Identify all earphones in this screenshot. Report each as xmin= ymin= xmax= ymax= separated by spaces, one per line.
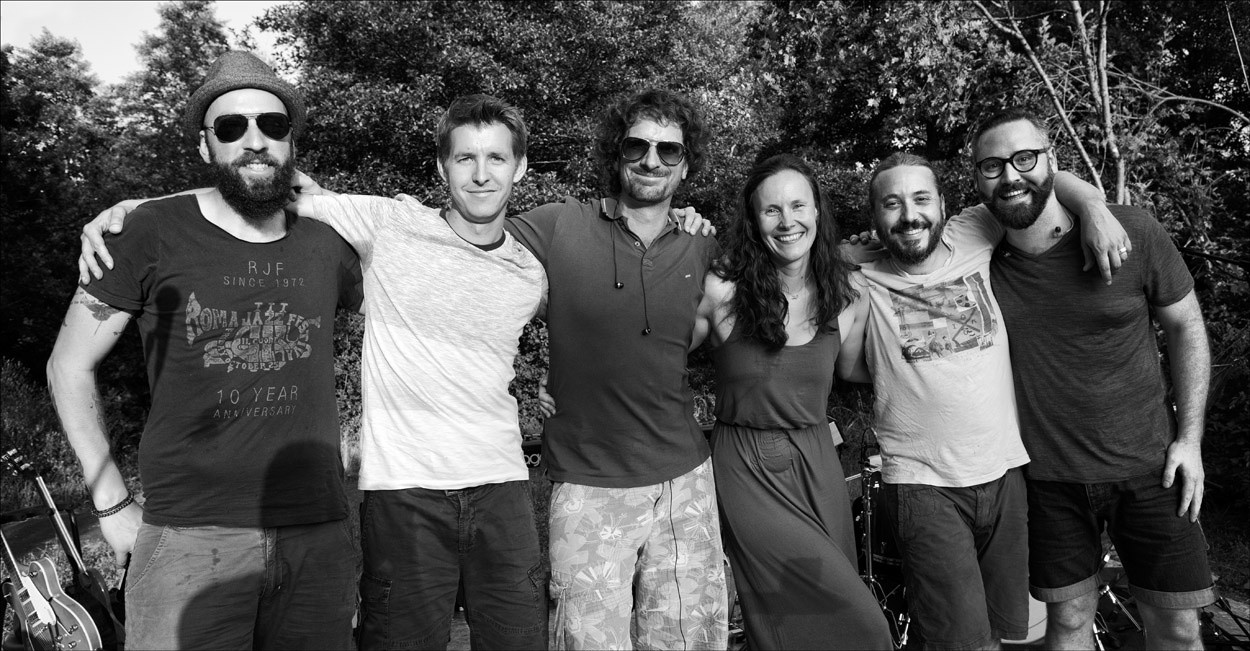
xmin=642 ymin=328 xmax=651 ymax=335
xmin=615 ymin=282 xmax=624 ymax=289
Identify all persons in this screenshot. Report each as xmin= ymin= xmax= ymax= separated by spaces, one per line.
xmin=841 ymin=105 xmax=1221 ymax=651
xmin=289 ymin=91 xmax=892 ymax=651
xmin=78 ymin=92 xmax=718 ymax=651
xmin=537 ymin=152 xmax=895 ymax=651
xmin=46 ymin=50 xmax=365 ymax=650
xmin=671 ymin=152 xmax=1133 ymax=651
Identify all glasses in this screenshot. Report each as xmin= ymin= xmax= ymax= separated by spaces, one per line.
xmin=976 ymin=148 xmax=1046 ymax=180
xmin=202 ymin=112 xmax=292 ymax=143
xmin=618 ymin=136 xmax=688 ymax=166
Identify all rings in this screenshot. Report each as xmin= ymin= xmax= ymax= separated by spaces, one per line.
xmin=1118 ymin=248 xmax=1127 ymax=254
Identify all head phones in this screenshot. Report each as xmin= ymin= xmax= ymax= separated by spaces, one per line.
xmin=999 ymin=201 xmax=1075 ymax=257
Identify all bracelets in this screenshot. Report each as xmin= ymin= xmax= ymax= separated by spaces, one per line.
xmin=90 ymin=489 xmax=134 ymax=518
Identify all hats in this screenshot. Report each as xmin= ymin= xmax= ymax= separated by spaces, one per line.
xmin=185 ymin=50 xmax=307 ymax=145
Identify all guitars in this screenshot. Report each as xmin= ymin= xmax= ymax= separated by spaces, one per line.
xmin=1 ymin=445 xmax=125 ymax=651
xmin=0 ymin=529 xmax=103 ymax=651
